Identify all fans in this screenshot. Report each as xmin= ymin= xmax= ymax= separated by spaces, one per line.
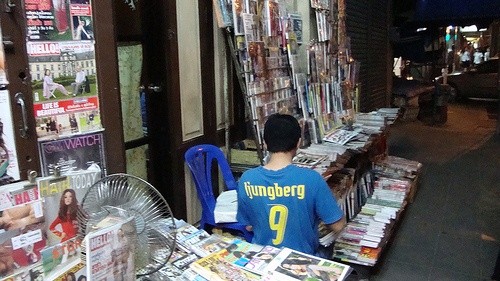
xmin=75 ymin=172 xmax=177 ymax=279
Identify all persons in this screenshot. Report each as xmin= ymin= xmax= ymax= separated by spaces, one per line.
xmin=50 ymin=189 xmax=90 ymax=243
xmin=43 ymin=65 xmax=86 ymax=98
xmin=448 ymin=45 xmax=490 ymax=72
xmin=279 ymin=257 xmax=343 ymax=281
xmin=236 ymin=113 xmax=346 ymax=257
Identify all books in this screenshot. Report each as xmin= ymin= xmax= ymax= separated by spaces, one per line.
xmin=0 ymin=178 xmax=354 ymax=281
xmin=222 ymin=0 xmax=423 ymax=267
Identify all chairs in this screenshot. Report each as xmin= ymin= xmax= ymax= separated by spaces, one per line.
xmin=186 ymin=144 xmax=254 ymax=244
